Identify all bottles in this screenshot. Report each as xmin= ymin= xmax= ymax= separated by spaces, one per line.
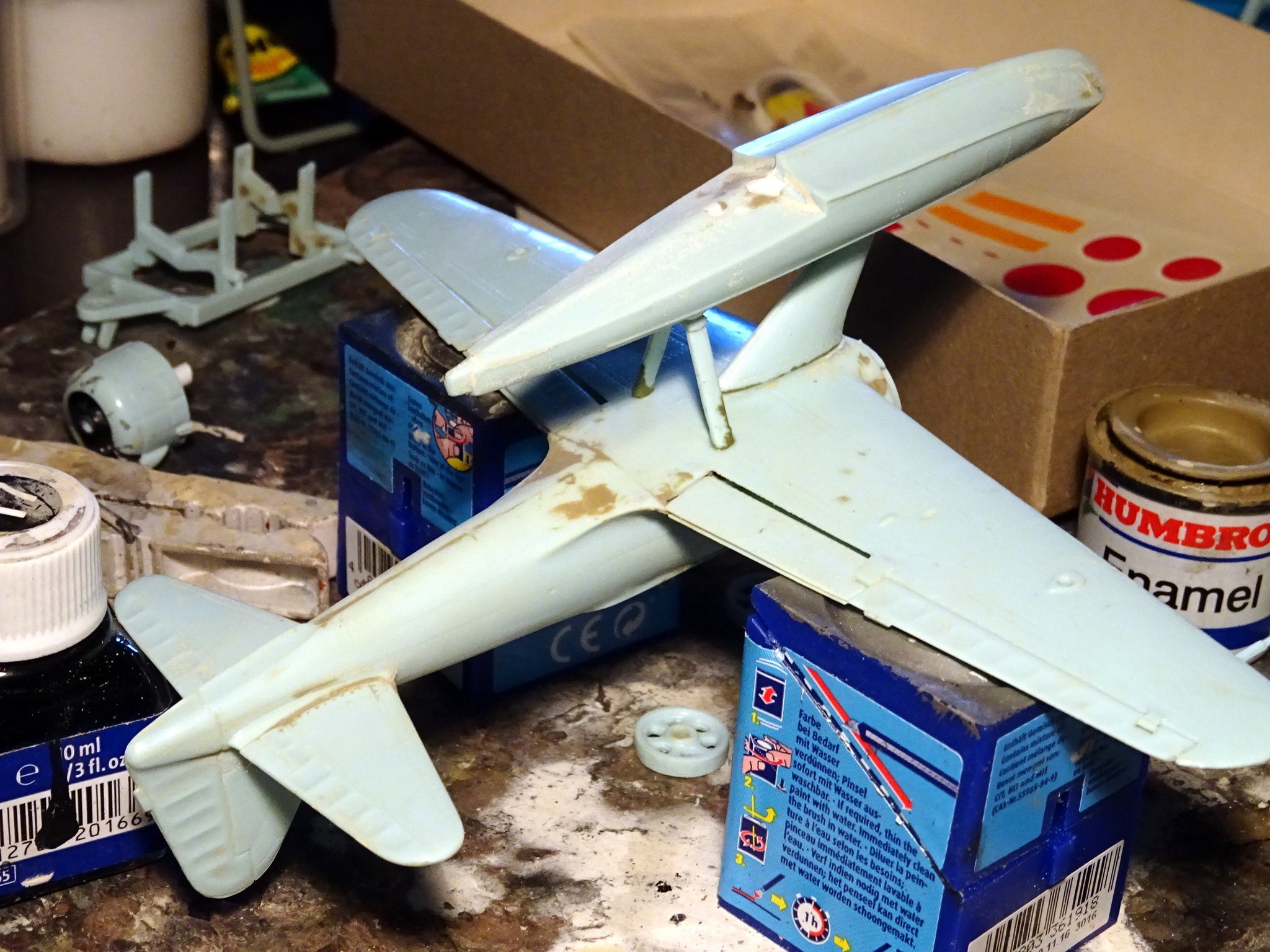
xmin=0 ymin=462 xmax=180 ymax=907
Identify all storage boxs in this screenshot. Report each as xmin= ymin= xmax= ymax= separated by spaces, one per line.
xmin=335 ymin=0 xmax=1270 ymax=515
xmin=335 ymin=300 xmax=716 ymax=696
xmin=717 ymin=572 xmax=1147 ymax=951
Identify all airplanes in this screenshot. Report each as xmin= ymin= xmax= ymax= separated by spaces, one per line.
xmin=111 ymin=47 xmax=1268 ymax=903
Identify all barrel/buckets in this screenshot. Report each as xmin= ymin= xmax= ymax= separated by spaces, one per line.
xmin=1075 ymin=381 xmax=1270 ymax=654
